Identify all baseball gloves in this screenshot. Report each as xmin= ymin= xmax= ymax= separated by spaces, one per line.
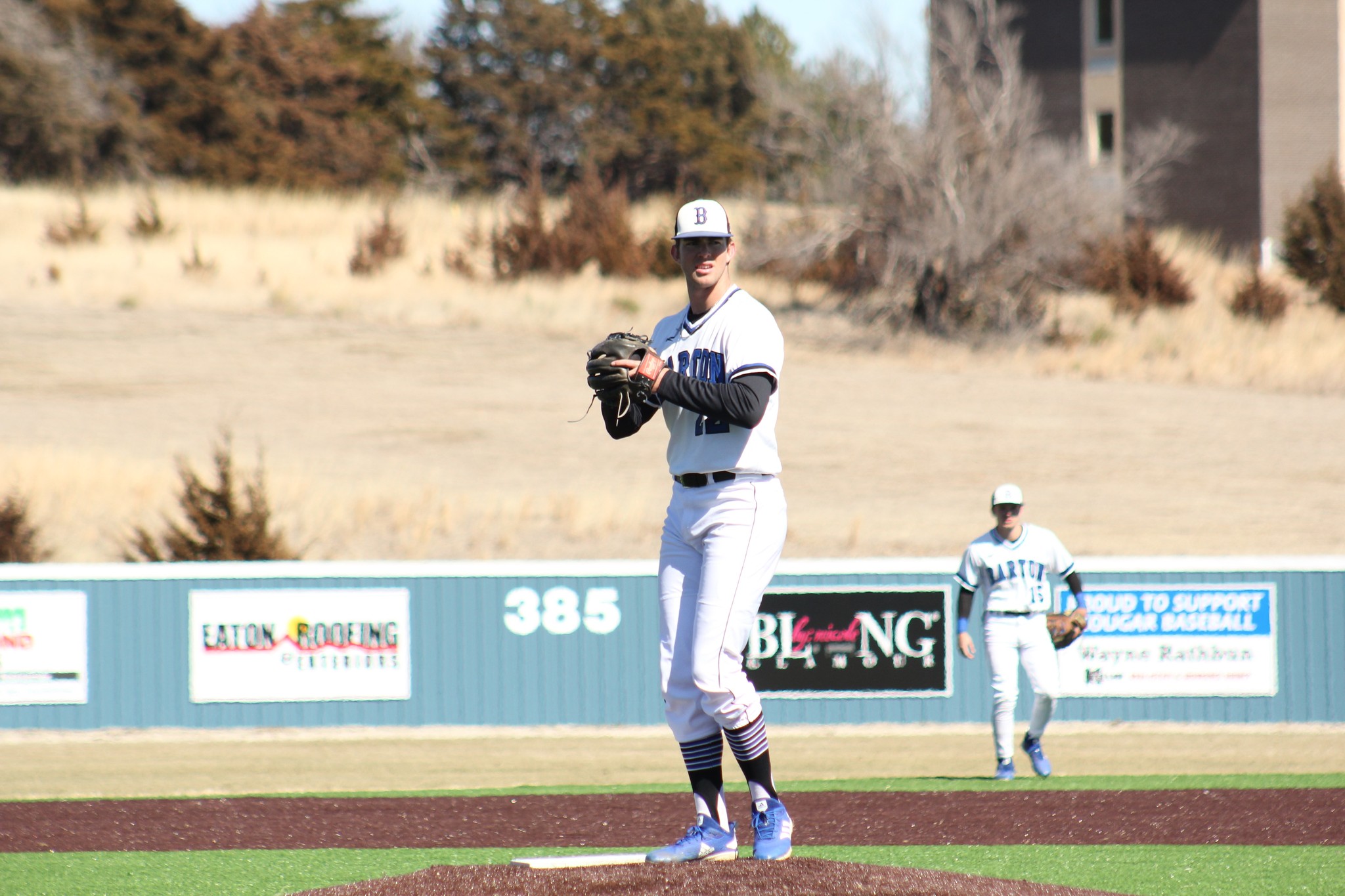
xmin=567 ymin=326 xmax=667 ymax=425
xmin=1046 ymin=610 xmax=1089 ymax=650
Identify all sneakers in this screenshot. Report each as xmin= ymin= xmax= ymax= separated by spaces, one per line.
xmin=752 ymin=798 xmax=792 ymax=861
xmin=1023 ymin=732 xmax=1052 ymax=777
xmin=996 ymin=759 xmax=1014 ymax=782
xmin=646 ymin=813 xmax=739 ymax=862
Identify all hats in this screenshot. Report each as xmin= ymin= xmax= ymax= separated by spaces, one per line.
xmin=993 ymin=483 xmax=1024 ymax=506
xmin=671 ymin=199 xmax=734 ymax=238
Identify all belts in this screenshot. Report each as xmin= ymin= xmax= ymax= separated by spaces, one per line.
xmin=991 ymin=611 xmax=1031 ymax=616
xmin=674 ymin=471 xmax=735 ymax=488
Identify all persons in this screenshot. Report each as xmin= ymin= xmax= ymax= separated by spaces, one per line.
xmin=569 ymin=200 xmax=796 ymax=866
xmin=955 ymin=483 xmax=1088 ymax=782
